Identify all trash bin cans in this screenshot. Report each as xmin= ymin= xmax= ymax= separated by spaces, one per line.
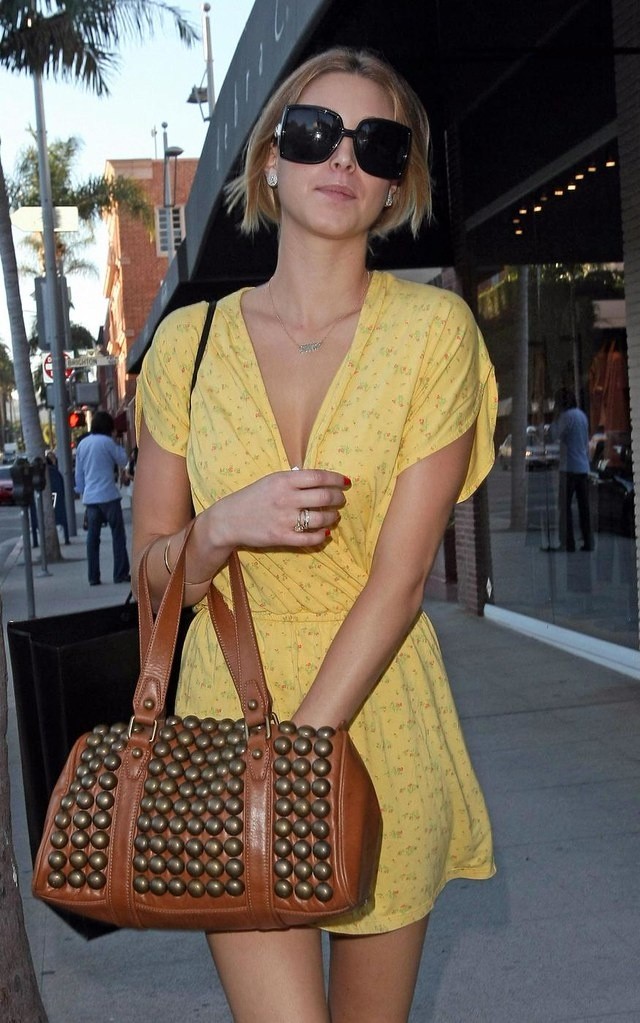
xmin=525 ymin=459 xmax=559 ymax=531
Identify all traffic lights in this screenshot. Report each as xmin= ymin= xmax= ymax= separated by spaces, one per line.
xmin=69 ymin=412 xmax=87 ymax=428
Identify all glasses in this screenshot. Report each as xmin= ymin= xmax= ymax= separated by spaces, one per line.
xmin=276 ymin=104 xmax=413 ymax=185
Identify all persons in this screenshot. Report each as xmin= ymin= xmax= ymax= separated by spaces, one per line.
xmin=131 ymin=50 xmax=497 ymax=1023
xmin=543 ymin=388 xmax=594 ymax=552
xmin=74 ymin=412 xmax=132 ymax=586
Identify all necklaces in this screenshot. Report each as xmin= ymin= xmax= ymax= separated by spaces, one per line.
xmin=268 ymin=269 xmax=369 ymax=355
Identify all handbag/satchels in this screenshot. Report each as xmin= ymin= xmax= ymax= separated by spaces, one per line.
xmin=8 ymin=601 xmax=202 ymax=943
xmin=27 ymin=518 xmax=389 ymax=939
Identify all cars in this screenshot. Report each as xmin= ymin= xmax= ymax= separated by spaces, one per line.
xmin=497 ymin=424 xmax=559 ymax=473
xmin=580 ymin=430 xmax=637 ymax=542
xmin=0 ymin=442 xmax=26 ymax=507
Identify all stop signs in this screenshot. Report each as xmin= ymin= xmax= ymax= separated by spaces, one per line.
xmin=43 ymin=352 xmax=74 ymax=381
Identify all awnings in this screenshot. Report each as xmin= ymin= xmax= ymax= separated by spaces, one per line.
xmin=45 ymin=382 xmax=98 ymax=449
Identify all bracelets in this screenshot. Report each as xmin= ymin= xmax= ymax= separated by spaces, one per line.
xmin=164 ymin=534 xmax=194 ymax=585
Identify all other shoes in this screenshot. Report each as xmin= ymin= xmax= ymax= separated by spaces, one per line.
xmin=113 ymin=574 xmax=131 ymax=584
xmin=89 ymin=580 xmax=101 ymax=586
xmin=552 ymin=542 xmax=576 ymax=552
xmin=579 ymin=543 xmax=595 ymax=551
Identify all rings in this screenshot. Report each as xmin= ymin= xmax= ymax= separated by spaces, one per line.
xmin=295 ymin=509 xmax=309 ymax=532
